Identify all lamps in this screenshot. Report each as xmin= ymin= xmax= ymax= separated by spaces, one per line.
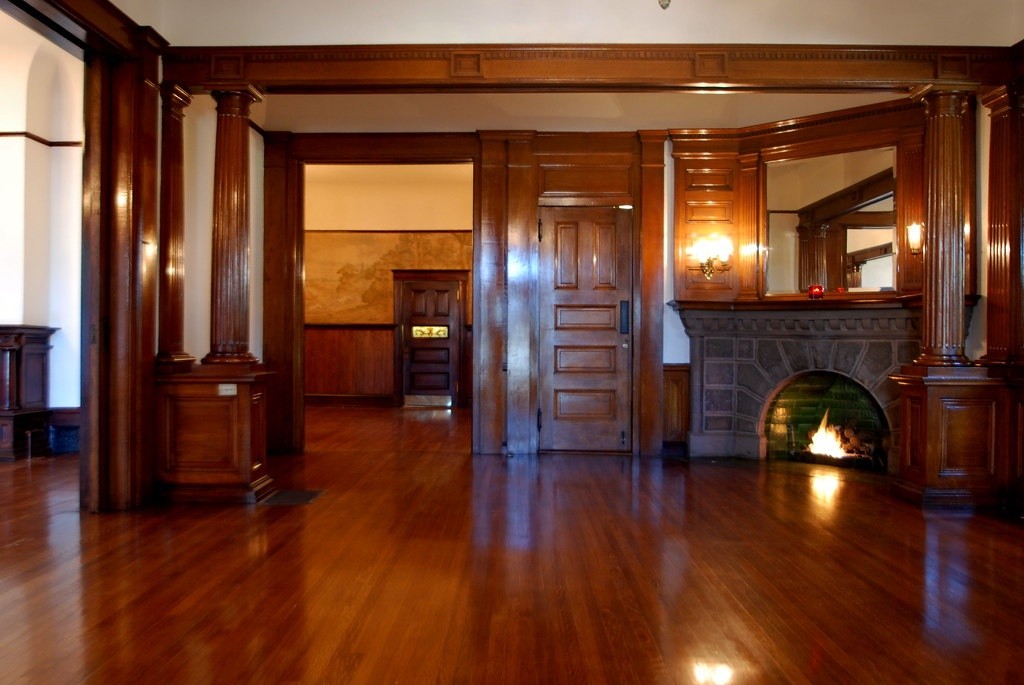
xmin=907 ymin=222 xmax=925 ymax=266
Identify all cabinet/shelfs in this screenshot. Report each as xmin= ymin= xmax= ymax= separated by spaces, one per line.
xmin=0 ymin=324 xmax=60 ymax=461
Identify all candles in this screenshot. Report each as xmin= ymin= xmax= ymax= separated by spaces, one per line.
xmin=808 ymin=285 xmax=824 ymax=300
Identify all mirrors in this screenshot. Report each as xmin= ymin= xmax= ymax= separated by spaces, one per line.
xmin=763 ymin=144 xmax=899 ymax=296
